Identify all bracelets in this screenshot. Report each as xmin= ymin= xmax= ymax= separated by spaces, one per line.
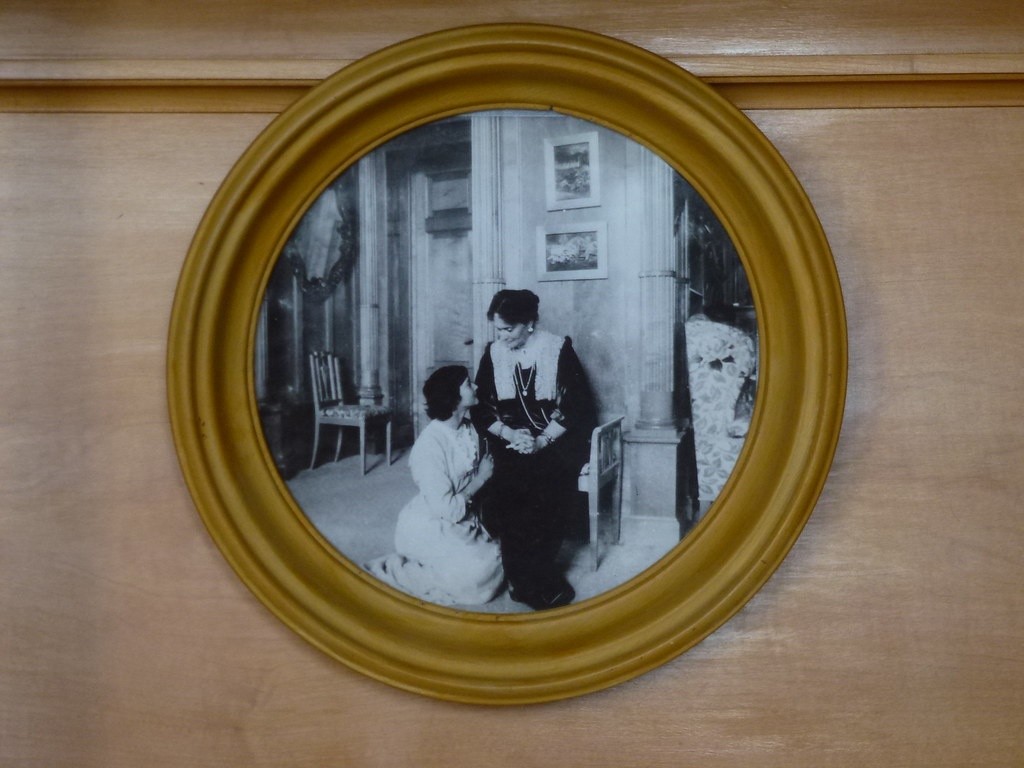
xmin=542 ymin=431 xmax=556 ymax=444
xmin=498 ymin=424 xmax=506 ymax=440
xmin=462 ymin=489 xmax=473 ymax=500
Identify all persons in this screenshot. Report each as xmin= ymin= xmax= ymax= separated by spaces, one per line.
xmin=561 ymin=170 xmax=578 ymax=192
xmin=470 ymin=289 xmax=596 ymax=610
xmin=365 ymin=366 xmax=504 ymax=607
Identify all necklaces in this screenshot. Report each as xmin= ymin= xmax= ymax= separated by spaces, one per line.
xmin=513 ymin=349 xmax=533 ymax=396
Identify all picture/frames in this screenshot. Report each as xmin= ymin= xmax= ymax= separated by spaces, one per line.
xmin=541 ymin=131 xmax=600 ymax=212
xmin=169 ymin=21 xmax=850 ymax=701
xmin=538 ymin=222 xmax=611 ymax=281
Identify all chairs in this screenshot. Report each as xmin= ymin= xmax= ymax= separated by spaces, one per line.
xmin=307 ymin=351 xmax=396 ymax=475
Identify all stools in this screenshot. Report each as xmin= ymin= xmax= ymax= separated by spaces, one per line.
xmin=570 ymin=415 xmax=628 ymax=571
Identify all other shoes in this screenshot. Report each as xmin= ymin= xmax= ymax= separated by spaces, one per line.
xmin=508 ymin=574 xmax=576 ymax=610
